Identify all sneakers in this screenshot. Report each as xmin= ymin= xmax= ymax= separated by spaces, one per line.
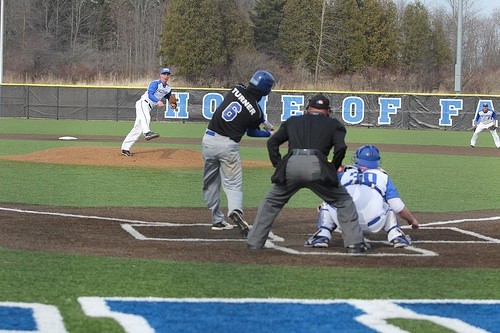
xmin=212 ymin=220 xmax=233 ymax=230
xmin=229 ymin=210 xmax=250 ymax=233
xmin=145 ymin=131 xmax=159 ymax=140
xmin=121 ymin=150 xmax=133 ymax=157
xmin=392 ymin=237 xmax=409 ymax=249
xmin=304 ymin=236 xmax=329 ymax=248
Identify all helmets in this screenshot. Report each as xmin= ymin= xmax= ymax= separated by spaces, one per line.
xmin=249 ymin=70 xmax=274 ymax=96
xmin=355 ymin=146 xmax=380 ymax=168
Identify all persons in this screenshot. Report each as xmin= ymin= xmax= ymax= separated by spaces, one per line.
xmin=202 ymin=70 xmax=276 ymax=234
xmin=122 ymin=68 xmax=177 ymax=156
xmin=307 ymin=145 xmax=419 ymax=249
xmin=470 ymin=103 xmax=500 ymax=148
xmin=246 ymin=95 xmax=371 ymax=253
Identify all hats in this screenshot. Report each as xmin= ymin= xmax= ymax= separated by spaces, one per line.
xmin=160 ymin=68 xmax=171 ymax=74
xmin=306 ymin=95 xmax=329 ymax=109
xmin=483 ymin=103 xmax=488 ymax=107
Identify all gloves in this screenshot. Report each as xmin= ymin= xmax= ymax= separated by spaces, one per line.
xmin=262 ymin=120 xmax=273 ymax=132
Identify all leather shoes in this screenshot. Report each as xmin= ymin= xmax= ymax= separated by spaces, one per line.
xmin=247 ymin=243 xmax=258 ymax=249
xmin=348 ymin=242 xmax=367 ymax=253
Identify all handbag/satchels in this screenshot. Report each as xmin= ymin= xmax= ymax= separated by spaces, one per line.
xmin=271 ymin=151 xmax=292 ymax=186
xmin=316 ymin=152 xmax=339 ymax=187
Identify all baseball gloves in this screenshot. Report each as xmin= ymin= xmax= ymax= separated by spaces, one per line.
xmin=170 ymin=94 xmax=176 ymax=104
xmin=488 ymin=125 xmax=498 ymax=131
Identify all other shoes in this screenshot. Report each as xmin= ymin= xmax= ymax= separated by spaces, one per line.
xmin=470 ymin=145 xmax=474 ymax=147
xmin=497 ymin=147 xmax=500 ymax=151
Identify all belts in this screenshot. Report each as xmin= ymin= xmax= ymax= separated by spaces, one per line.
xmin=206 ymin=131 xmax=215 ymax=136
xmin=289 ymin=148 xmax=318 ymax=155
xmin=145 ymin=100 xmax=152 ymax=108
xmin=367 ymin=216 xmax=380 ymax=226
